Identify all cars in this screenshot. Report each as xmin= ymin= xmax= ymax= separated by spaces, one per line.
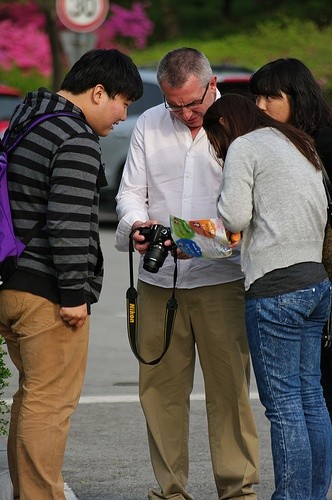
xmin=0 ymin=82 xmax=22 ymax=135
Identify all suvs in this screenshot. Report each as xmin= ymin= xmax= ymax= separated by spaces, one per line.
xmin=97 ymin=67 xmax=255 ymax=225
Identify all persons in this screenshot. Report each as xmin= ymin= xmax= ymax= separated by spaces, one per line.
xmin=115 ymin=48 xmax=260 ymax=500
xmin=202 ymin=95 xmax=332 ymax=500
xmin=0 ymin=49 xmax=143 ymax=500
xmin=252 ymin=58 xmax=332 ymax=421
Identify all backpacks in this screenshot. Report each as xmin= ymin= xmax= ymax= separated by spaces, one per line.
xmin=0 ymin=111 xmax=108 ymax=288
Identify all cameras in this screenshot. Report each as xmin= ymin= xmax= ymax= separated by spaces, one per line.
xmin=139 ymin=224 xmax=176 ymax=273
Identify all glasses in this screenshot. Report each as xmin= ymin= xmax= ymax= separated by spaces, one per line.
xmin=163 ymin=83 xmax=209 ymax=111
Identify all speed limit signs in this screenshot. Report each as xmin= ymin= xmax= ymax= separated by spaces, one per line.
xmin=55 ymin=0 xmax=109 ymax=33
